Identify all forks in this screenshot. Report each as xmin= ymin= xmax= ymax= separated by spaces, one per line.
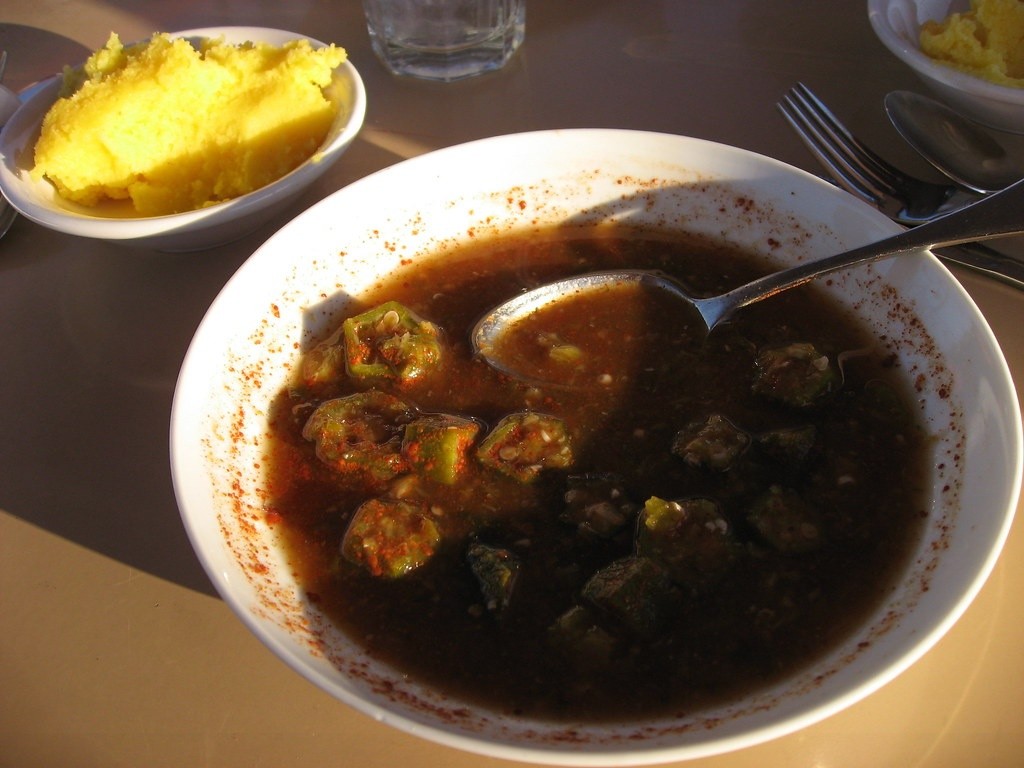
xmin=775 ymin=80 xmax=987 ymax=227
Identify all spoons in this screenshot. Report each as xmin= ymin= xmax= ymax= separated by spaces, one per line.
xmin=885 ymin=90 xmax=1024 ymax=195
xmin=474 ymin=179 xmax=1024 ymax=388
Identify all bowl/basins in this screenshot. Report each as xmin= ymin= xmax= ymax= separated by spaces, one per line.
xmin=867 ymin=0 xmax=1024 ymax=129
xmin=170 ymin=129 xmax=1024 ymax=768
xmin=0 ymin=26 xmax=367 ymax=252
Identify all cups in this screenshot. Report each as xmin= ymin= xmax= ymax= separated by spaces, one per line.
xmin=362 ymin=0 xmax=527 ymax=83
